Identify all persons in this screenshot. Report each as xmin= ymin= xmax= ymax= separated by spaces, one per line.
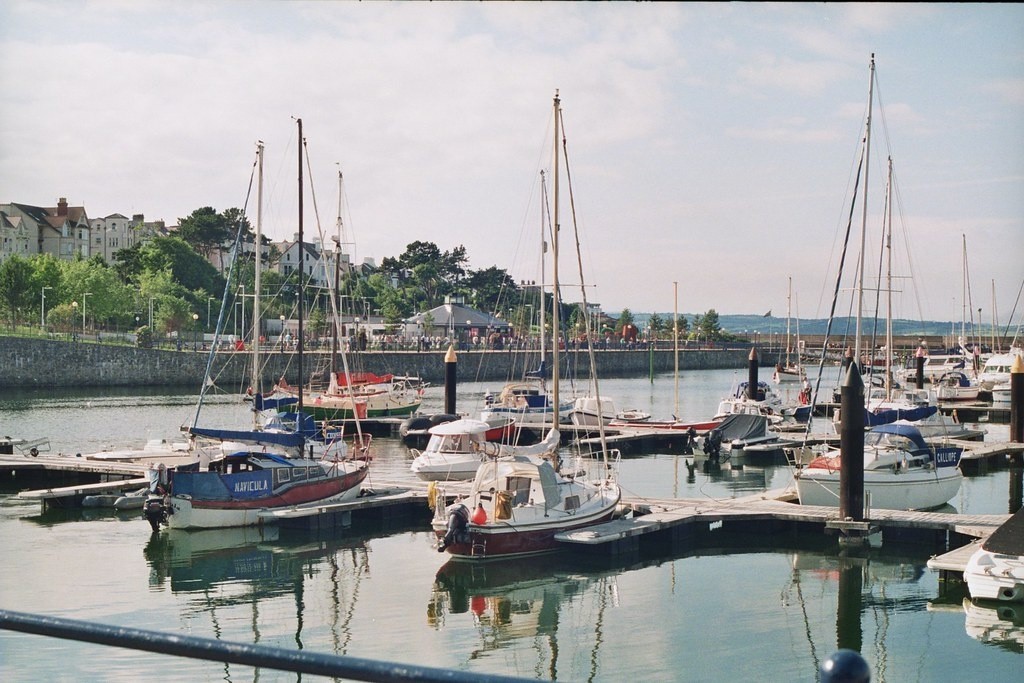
xmin=606 ymin=336 xmax=612 ymax=348
xmin=802 ymin=376 xmax=812 ymax=404
xmin=279 ymin=332 xmax=284 ymax=352
xmin=345 ymin=339 xmax=351 ymax=353
xmin=284 ymin=332 xmax=293 ymax=351
xmin=620 ymin=336 xmax=626 ymax=350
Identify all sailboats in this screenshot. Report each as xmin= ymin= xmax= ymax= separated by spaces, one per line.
xmin=185 ymin=140 xmax=348 ymax=460
xmin=791 ymin=53 xmax=963 ymax=506
xmin=482 ymin=268 xmax=576 ymax=427
xmin=690 ymin=234 xmax=1024 ymax=466
xmin=261 ymin=160 xmax=426 ymax=426
xmin=410 ymin=170 xmax=555 ymax=483
xmin=163 ymin=114 xmax=370 ymax=528
xmin=430 ymin=88 xmax=631 ymax=561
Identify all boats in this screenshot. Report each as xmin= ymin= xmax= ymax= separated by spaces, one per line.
xmin=568 ymin=394 xmax=614 ymax=427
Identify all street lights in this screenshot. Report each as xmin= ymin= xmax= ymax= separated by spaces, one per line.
xmin=134 ymin=316 xmax=140 ymax=330
xmin=545 ymin=320 xmax=798 ymax=348
xmin=192 ymin=313 xmax=199 ymax=351
xmin=466 ymin=319 xmax=472 ymax=352
xmin=354 ymin=316 xmax=361 ymax=352
xmin=416 ymin=320 xmax=426 ymax=354
xmin=71 ymin=301 xmax=79 ymax=340
xmin=148 ymin=296 xmax=156 ymax=334
xmin=207 ymin=297 xmax=216 ymax=330
xmin=40 ymin=285 xmax=53 ymax=326
xmin=280 ymin=315 xmax=286 ymax=353
xmin=507 ymin=321 xmax=513 ymax=350
xmin=239 ymin=285 xmax=245 ymax=342
xmin=82 ymin=291 xmax=94 ymax=335
